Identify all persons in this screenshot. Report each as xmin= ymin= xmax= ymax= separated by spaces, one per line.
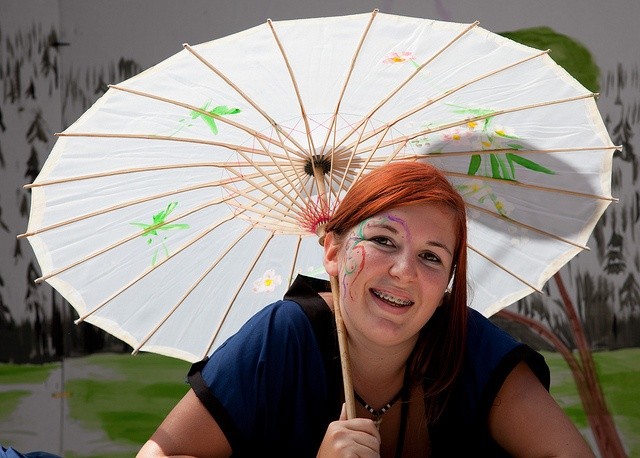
xmin=136 ymin=161 xmax=600 ymax=457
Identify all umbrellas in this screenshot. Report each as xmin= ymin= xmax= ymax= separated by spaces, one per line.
xmin=15 ymin=9 xmax=623 ymax=422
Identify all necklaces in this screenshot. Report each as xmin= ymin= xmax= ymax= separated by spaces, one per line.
xmin=354 ymin=384 xmax=407 ymax=431
